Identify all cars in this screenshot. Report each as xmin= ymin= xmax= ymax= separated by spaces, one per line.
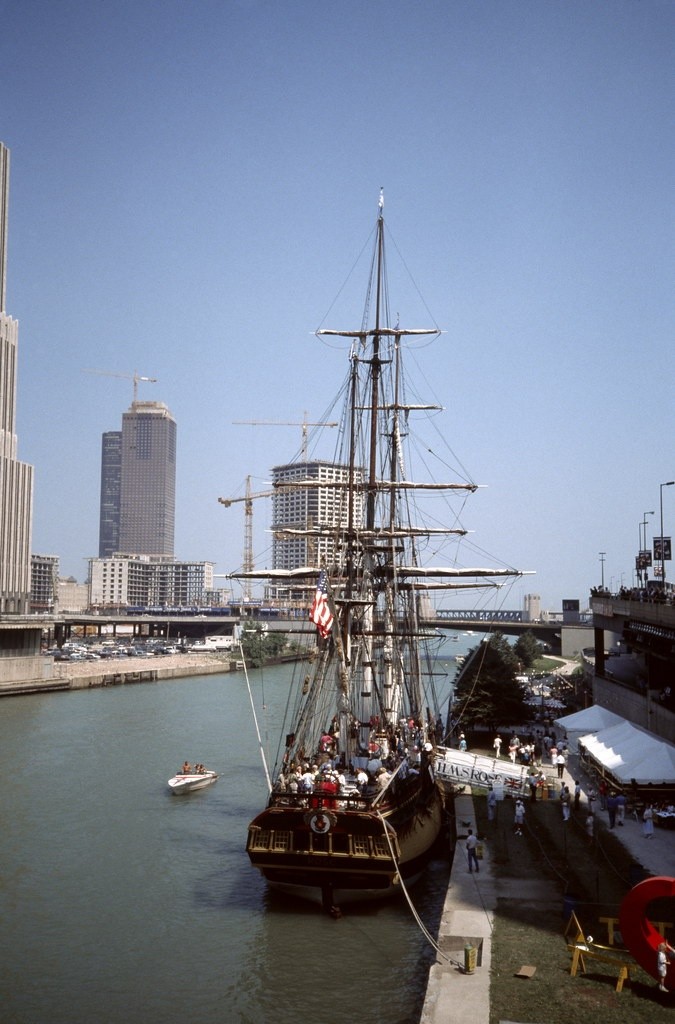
xmin=44 ymin=636 xmax=234 ymax=660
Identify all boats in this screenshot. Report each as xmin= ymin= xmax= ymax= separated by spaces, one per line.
xmin=168 ymin=770 xmax=220 ymax=795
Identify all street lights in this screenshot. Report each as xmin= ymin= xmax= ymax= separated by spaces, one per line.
xmin=644 ymin=511 xmax=655 ymax=588
xmin=639 ymin=522 xmax=649 ymax=588
xmin=659 ymin=481 xmax=675 ymax=590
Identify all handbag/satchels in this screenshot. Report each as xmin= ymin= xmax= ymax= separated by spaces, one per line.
xmin=562 ymin=802 xmax=567 ymax=806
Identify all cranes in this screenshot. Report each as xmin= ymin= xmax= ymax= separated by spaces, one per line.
xmin=218 ymin=474 xmax=319 ymax=598
xmin=231 ymin=408 xmax=339 ymax=463
xmin=84 ymin=366 xmax=157 ymax=412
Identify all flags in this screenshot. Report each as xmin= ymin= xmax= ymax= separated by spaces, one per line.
xmin=309 ymin=569 xmax=333 ymax=638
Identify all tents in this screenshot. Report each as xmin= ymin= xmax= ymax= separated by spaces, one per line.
xmin=554 ymin=704 xmax=675 ymax=790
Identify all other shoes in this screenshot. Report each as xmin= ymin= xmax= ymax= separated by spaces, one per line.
xmin=519 ymin=831 xmax=522 ymax=836
xmin=563 ymin=818 xmax=568 ymax=820
xmin=515 ymin=831 xmax=519 ymax=834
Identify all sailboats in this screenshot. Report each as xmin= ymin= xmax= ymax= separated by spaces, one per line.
xmin=220 ymin=188 xmax=537 ymax=907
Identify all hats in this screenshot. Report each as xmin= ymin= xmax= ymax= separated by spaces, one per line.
xmin=610 ymin=792 xmax=616 ymax=796
xmin=460 ymin=734 xmax=465 ymax=738
xmin=516 ymin=800 xmax=520 ymax=803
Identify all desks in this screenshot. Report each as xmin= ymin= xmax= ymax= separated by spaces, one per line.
xmin=656 ymin=813 xmax=675 ymax=829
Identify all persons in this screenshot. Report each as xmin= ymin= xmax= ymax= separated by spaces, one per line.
xmin=493 ymin=735 xmax=502 ymax=758
xmin=608 ymin=793 xmax=625 ymax=829
xmin=449 ymin=712 xmax=468 ymax=751
xmin=587 ymin=786 xmax=593 ymax=811
xmin=586 ymin=811 xmax=593 ymax=846
xmin=279 ymin=712 xmax=443 ymax=807
xmin=560 ymin=781 xmax=580 ymax=821
xmin=466 ymin=830 xmax=479 ymax=873
xmin=487 ymin=787 xmax=495 ymax=821
xmin=643 ymin=805 xmax=655 ymax=839
xmin=590 ymin=584 xmax=675 ymax=606
xmin=508 ymin=730 xmax=568 ymax=802
xmin=196 ymin=763 xmax=207 ymax=774
xmin=657 ymin=943 xmax=671 ymax=992
xmin=535 ymin=708 xmax=562 ymax=721
xmin=512 ymin=797 xmax=525 ymax=836
xmin=182 ymin=761 xmax=192 ymax=774
